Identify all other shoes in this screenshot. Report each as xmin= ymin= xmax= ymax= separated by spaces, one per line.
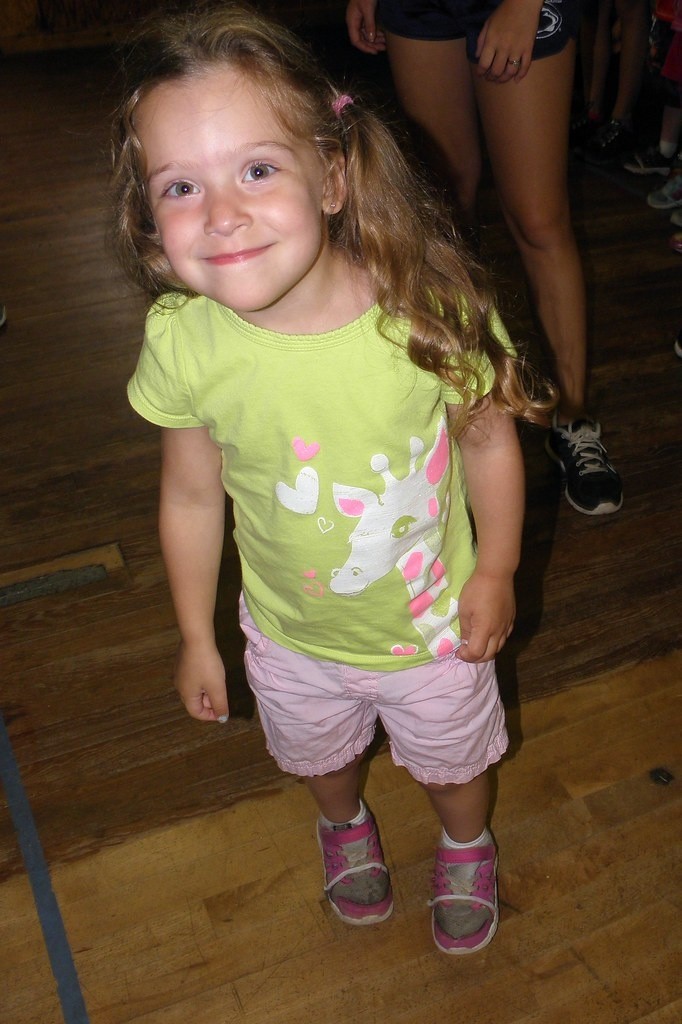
xmin=669 ymin=232 xmax=682 ymax=253
xmin=674 ymin=331 xmax=682 ymax=358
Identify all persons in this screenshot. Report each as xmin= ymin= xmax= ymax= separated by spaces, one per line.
xmin=344 ymin=0 xmax=681 ymax=517
xmin=106 ymin=0 xmax=556 ymax=958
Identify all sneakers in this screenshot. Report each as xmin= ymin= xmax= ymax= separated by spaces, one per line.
xmin=625 ymin=146 xmax=670 ymax=177
xmin=316 ymin=810 xmax=394 ymax=925
xmin=647 ymin=175 xmax=682 ymax=209
xmin=428 ymin=832 xmax=498 ymax=955
xmin=544 ymin=407 xmax=623 ymax=515
xmin=569 ymin=117 xmax=640 ymax=165
xmin=671 ymin=209 xmax=682 ymax=226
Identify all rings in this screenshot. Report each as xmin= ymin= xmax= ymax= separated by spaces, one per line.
xmin=509 ymin=58 xmax=520 ymax=65
